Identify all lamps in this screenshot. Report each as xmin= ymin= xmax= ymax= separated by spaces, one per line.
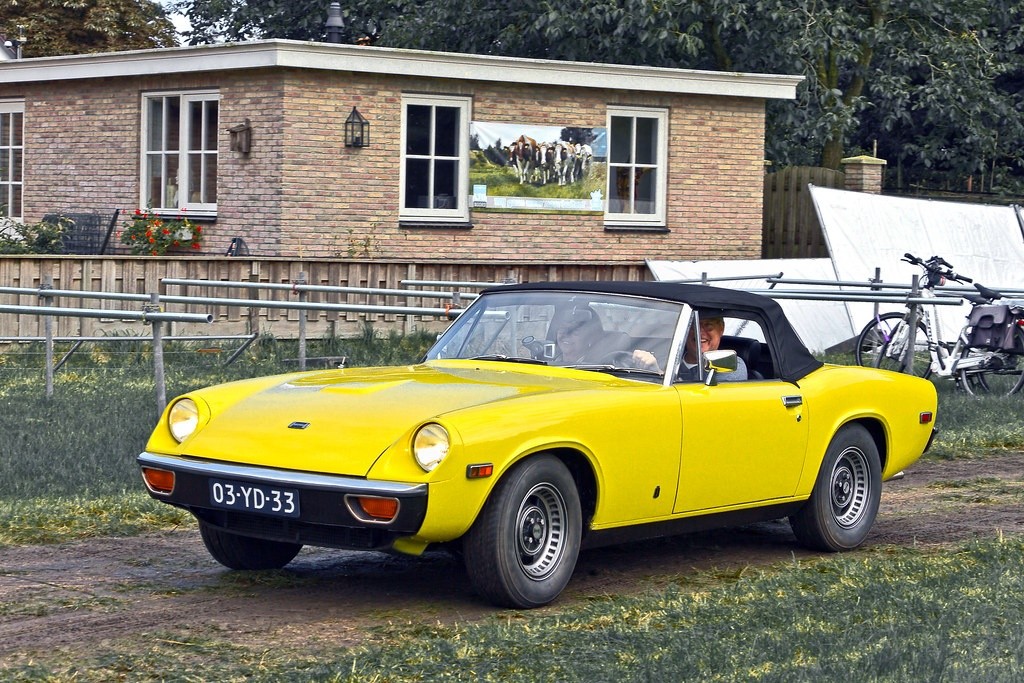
xmin=345 ymin=106 xmax=370 ymax=148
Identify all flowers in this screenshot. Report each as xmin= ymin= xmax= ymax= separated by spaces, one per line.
xmin=122 ymin=209 xmax=204 ymax=256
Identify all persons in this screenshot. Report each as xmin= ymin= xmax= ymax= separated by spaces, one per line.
xmin=518 ymin=305 xmax=602 ymax=364
xmin=631 ymin=309 xmax=747 ymax=382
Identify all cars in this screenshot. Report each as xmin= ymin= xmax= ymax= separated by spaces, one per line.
xmin=141 ymin=280 xmax=938 ymax=610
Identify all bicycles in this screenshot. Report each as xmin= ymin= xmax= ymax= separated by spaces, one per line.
xmin=854 ymin=252 xmax=1024 ymax=399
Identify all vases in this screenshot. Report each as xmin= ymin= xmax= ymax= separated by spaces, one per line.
xmin=148 ymin=226 xmax=160 ymax=235
xmin=173 ymin=227 xmax=192 ymax=241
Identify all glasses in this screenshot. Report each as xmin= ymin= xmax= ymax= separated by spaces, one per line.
xmin=691 ymin=321 xmax=718 ymax=332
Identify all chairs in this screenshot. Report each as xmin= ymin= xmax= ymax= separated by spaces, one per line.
xmin=717 ymin=335 xmax=765 ymax=381
